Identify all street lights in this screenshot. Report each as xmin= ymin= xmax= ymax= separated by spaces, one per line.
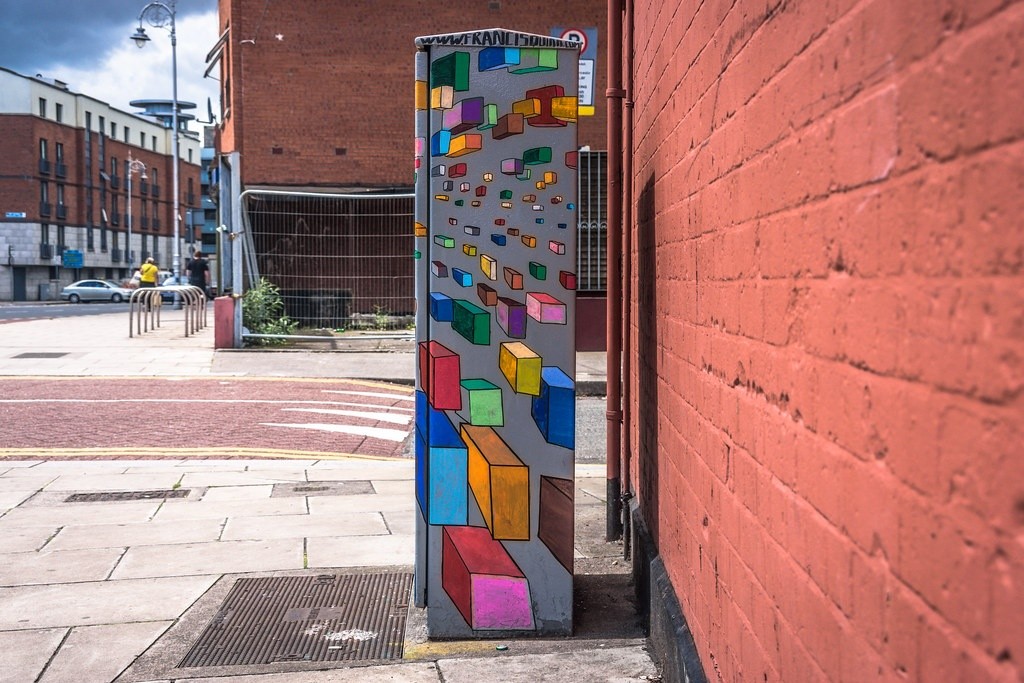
xmin=129 ymin=0 xmax=182 ymax=310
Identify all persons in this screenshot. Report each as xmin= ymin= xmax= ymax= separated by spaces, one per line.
xmin=139 ymin=256 xmax=160 ymax=312
xmin=186 ymin=250 xmax=210 ymax=294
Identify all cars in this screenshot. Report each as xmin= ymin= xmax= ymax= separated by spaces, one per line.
xmin=60 ymin=270 xmax=189 ymax=304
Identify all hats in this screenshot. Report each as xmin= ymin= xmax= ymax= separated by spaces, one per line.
xmin=147 ymin=257 xmax=154 ymax=262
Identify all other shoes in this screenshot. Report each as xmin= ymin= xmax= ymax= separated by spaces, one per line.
xmin=146 ymin=309 xmax=151 ymax=312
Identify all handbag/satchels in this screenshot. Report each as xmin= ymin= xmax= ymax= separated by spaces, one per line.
xmin=129 ymin=271 xmax=141 ymax=285
